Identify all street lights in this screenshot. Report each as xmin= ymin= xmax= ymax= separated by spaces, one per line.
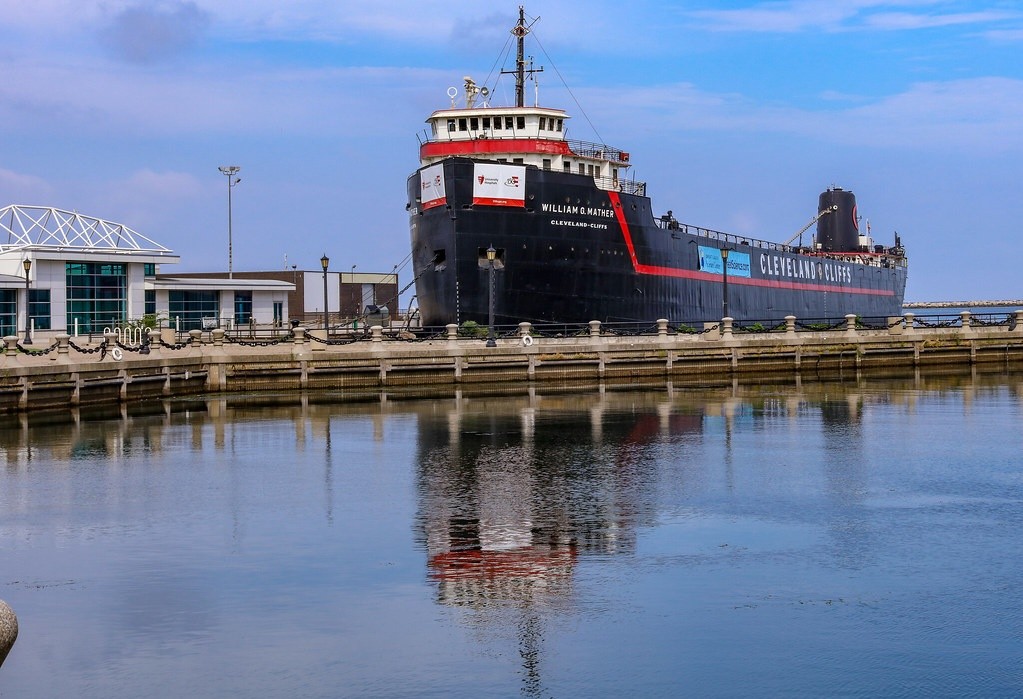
xmin=217 ymin=164 xmax=242 ymax=278
xmin=22 ymin=258 xmax=33 ymax=345
xmin=291 ymin=265 xmax=298 ymax=282
xmin=320 ymin=254 xmax=329 ymax=331
xmin=351 ymin=264 xmax=357 ymax=287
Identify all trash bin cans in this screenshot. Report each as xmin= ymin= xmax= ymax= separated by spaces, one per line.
xmin=363 ymin=305 xmax=389 ymax=326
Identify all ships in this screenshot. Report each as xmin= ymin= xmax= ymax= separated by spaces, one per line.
xmin=408 ymin=6 xmax=911 ymax=337
